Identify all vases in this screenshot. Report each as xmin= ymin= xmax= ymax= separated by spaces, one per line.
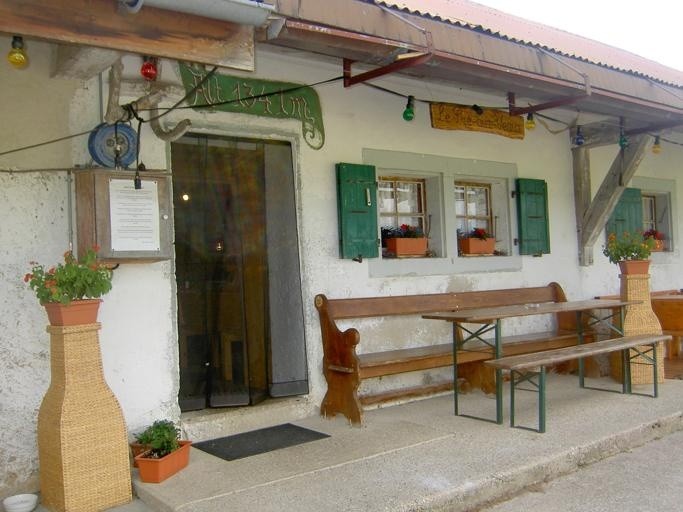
xmin=45 ymin=300 xmax=104 ymax=326
xmin=456 ymin=237 xmax=498 ymax=255
xmin=384 ymin=237 xmax=428 ymax=256
xmin=619 ymin=259 xmax=650 ymax=275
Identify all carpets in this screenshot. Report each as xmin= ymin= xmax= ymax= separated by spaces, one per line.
xmin=189 ymin=423 xmax=330 ymax=463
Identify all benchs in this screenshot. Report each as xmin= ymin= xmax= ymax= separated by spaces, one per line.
xmin=314 ymin=283 xmax=601 ymax=430
xmin=486 ymin=333 xmax=672 ymax=434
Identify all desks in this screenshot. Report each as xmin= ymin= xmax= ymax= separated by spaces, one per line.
xmin=421 ymin=297 xmax=645 ymax=423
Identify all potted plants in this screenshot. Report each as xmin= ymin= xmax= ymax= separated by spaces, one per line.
xmin=129 ymin=420 xmax=192 ymax=482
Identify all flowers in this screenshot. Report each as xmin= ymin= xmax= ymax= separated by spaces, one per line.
xmin=598 ymin=228 xmax=665 ymax=263
xmin=25 ymin=240 xmax=119 ymax=306
xmin=462 ymin=227 xmax=496 ymax=239
xmin=388 ymin=223 xmax=425 ymax=239
